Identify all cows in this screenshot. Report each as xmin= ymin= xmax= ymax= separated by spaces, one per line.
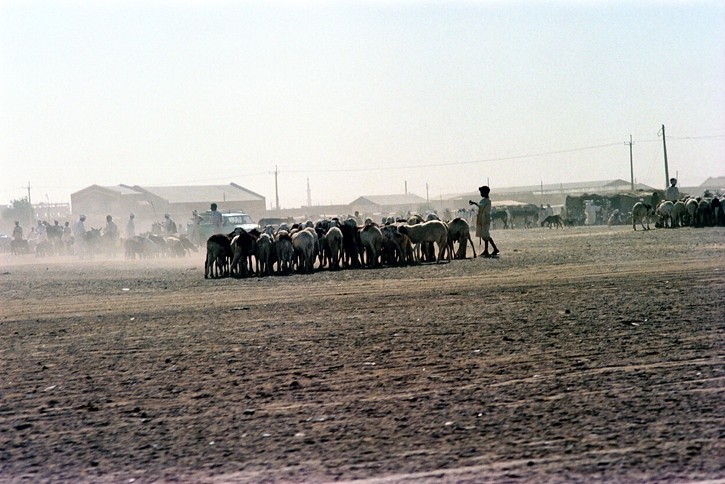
xmin=1 ymin=193 xmax=725 ymax=278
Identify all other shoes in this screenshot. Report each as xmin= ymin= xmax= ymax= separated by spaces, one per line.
xmin=490 ymin=250 xmax=500 ymax=255
xmin=481 ymin=252 xmax=489 ymax=255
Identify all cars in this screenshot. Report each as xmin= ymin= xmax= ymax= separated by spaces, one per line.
xmin=258 ymin=218 xmax=282 ymax=229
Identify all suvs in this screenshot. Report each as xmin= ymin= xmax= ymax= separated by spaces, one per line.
xmin=199 ymin=210 xmax=261 ymax=235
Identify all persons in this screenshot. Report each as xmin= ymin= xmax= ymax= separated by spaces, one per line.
xmin=12 ymin=221 xmax=23 ymax=241
xmin=546 ymin=204 xmax=554 ymax=217
xmin=76 ymin=214 xmax=87 ymax=259
xmin=104 ymin=215 xmax=118 ymax=259
xmin=35 ymin=219 xmax=74 ymax=248
xmin=665 ymin=178 xmax=679 ymax=204
xmin=190 ymin=210 xmax=204 ymax=248
xmin=161 ymin=213 xmax=173 ymax=232
xmin=561 ymin=207 xmax=566 ymax=218
xmin=651 ymin=192 xmax=659 ymax=210
xmin=178 ymin=224 xmax=186 ymax=233
xmin=126 ymin=214 xmax=135 ymax=238
xmin=354 ymin=211 xmax=363 ymax=225
xmin=469 ymin=186 xmax=500 ymax=256
xmin=210 ymin=203 xmax=224 ymax=234
xmin=539 ymin=204 xmax=545 ymax=211
xmin=28 ymin=226 xmax=37 ymax=240
